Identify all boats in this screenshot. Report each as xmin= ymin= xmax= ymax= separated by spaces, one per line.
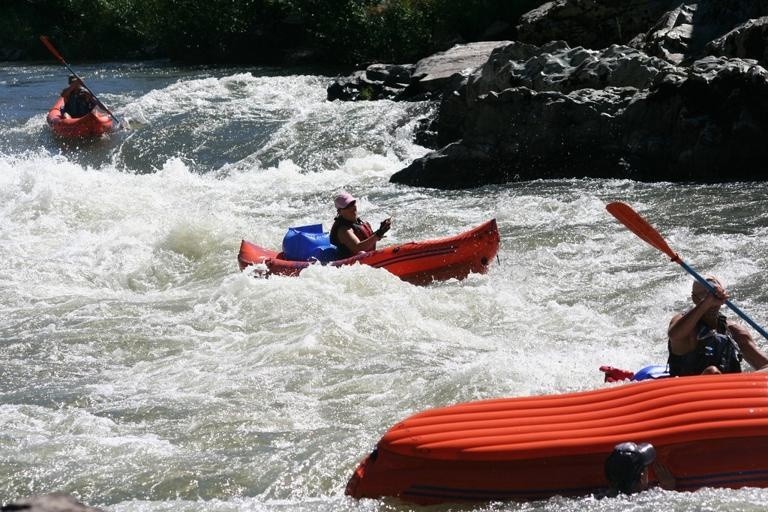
xmin=344 ymin=364 xmax=767 ymax=502
xmin=236 ymin=216 xmax=500 ymax=291
xmin=46 ymin=96 xmax=113 ymax=138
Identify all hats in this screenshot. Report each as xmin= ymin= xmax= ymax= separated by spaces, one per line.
xmin=692 ymin=275 xmax=721 ymax=298
xmin=334 ymin=193 xmax=357 ymax=209
xmin=69 ymin=74 xmax=77 ymax=83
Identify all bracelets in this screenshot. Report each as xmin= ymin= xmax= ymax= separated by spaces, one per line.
xmin=375 ymin=229 xmax=384 ymax=238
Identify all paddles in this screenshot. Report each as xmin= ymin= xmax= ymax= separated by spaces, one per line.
xmin=38 ymin=36 xmax=117 ymax=122
xmin=604 ymin=201 xmax=768 ymax=341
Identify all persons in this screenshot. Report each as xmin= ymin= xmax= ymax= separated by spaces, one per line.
xmin=607 ymin=442 xmax=658 ymax=495
xmin=329 ymin=192 xmax=392 ymax=260
xmin=666 ymin=275 xmax=768 ymax=375
xmin=60 ymin=73 xmax=98 ymax=119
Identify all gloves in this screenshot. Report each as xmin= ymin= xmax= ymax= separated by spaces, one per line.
xmin=376 ymin=221 xmax=391 ymax=236
